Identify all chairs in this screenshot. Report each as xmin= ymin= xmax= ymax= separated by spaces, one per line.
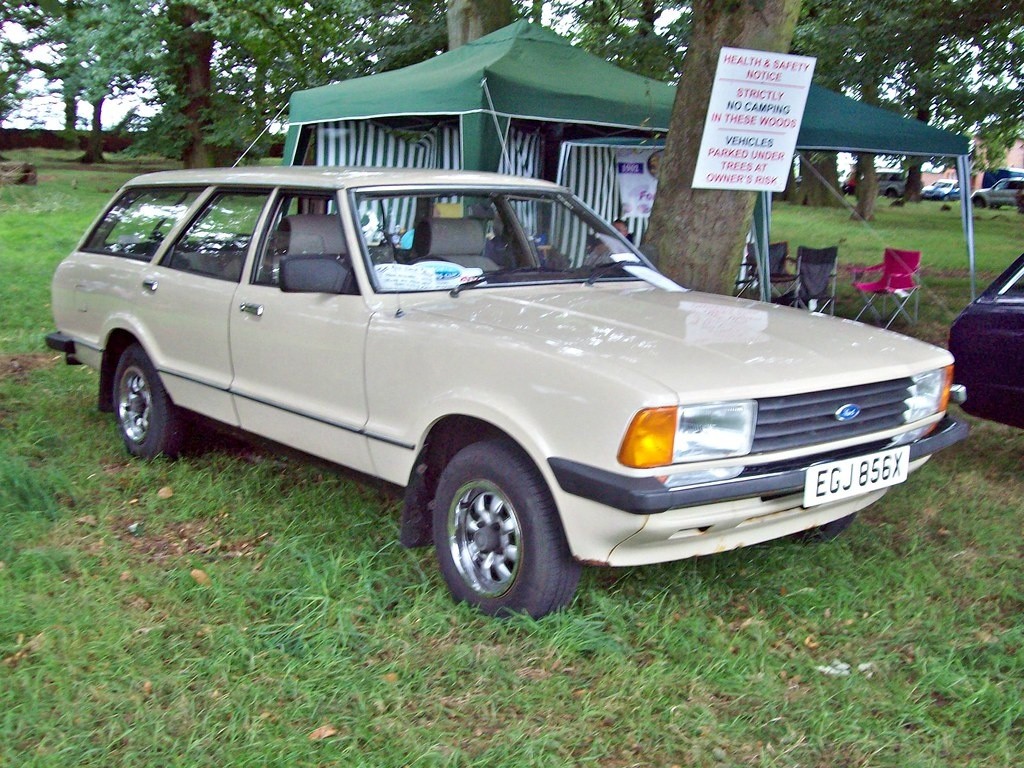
xmin=584 ymin=234 xmax=633 ymax=251
xmin=740 ymin=241 xmax=838 ymax=317
xmin=274 ymin=213 xmax=358 ymax=293
xmin=851 ymin=248 xmax=923 ymax=329
xmin=410 ymin=217 xmax=501 ymax=274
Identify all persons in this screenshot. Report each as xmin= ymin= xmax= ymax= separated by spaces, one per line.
xmin=582 ymin=219 xmax=628 ymax=268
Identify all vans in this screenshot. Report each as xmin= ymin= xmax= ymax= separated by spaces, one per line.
xmin=982 ymin=168 xmax=1024 ymax=188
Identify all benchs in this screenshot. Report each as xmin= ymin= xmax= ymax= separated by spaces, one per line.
xmin=174 ymin=248 xmax=283 ymax=284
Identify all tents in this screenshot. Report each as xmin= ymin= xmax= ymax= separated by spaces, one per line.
xmin=280 ymin=20 xmax=765 ymax=306
xmin=751 ymin=81 xmax=976 ymax=305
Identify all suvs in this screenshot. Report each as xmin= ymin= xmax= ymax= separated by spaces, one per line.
xmin=841 ymin=169 xmax=860 ymax=197
xmin=876 ymin=173 xmax=907 ymax=198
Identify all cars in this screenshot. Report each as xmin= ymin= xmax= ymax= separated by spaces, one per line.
xmin=920 ymin=179 xmax=960 ymax=201
xmin=971 ymin=177 xmax=1024 ymax=208
xmin=948 ymin=253 xmax=1024 ymax=433
xmin=44 ymin=167 xmax=969 ymax=618
xmin=781 ymin=175 xmax=803 ymax=201
